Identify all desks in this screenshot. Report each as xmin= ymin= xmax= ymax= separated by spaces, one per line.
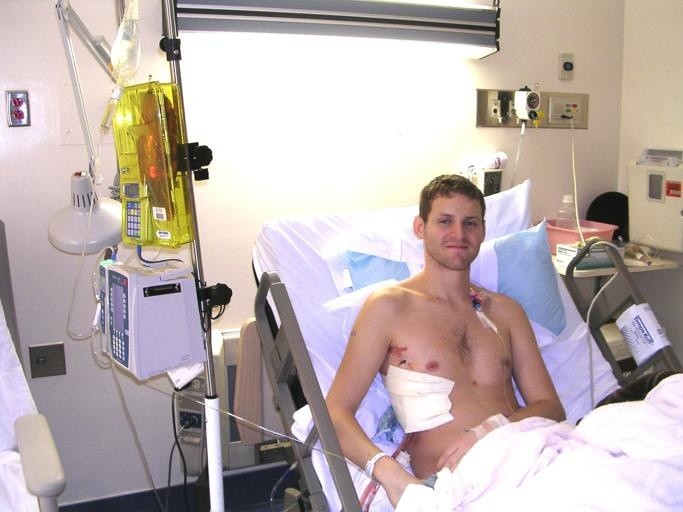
xmin=552 ymin=246 xmax=679 ymax=364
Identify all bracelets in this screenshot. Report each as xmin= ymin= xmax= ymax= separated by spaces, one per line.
xmin=364 ymin=452 xmax=385 ymax=478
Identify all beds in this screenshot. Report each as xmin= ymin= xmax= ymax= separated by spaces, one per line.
xmin=248 ymin=178 xmax=683 ymax=512
xmin=0 ymin=220 xmax=67 ymax=511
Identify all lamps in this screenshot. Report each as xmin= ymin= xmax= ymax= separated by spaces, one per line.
xmin=160 ymin=0 xmax=501 ymax=59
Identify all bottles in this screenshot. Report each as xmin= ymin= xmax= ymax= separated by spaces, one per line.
xmin=554 ymin=194 xmax=578 ymax=229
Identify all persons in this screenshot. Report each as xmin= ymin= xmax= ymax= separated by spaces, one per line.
xmin=323 ymin=173 xmax=567 ymax=512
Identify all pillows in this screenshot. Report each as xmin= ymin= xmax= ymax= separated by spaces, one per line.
xmin=344 ymin=217 xmax=566 ymax=343
